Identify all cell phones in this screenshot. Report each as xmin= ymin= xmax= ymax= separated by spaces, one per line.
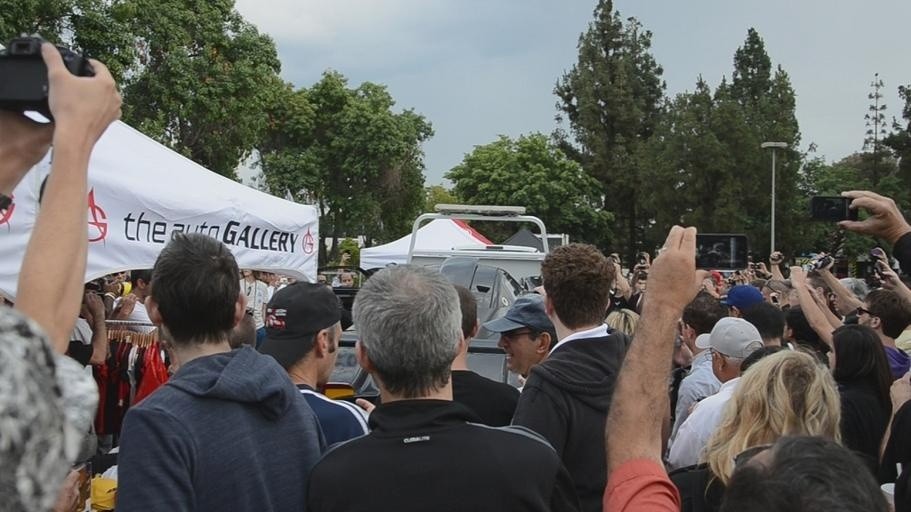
xmin=857 ymin=261 xmax=884 ymax=278
xmin=751 ymin=265 xmax=760 ymax=269
xmin=638 ymin=265 xmax=646 ymax=270
xmin=694 ymin=234 xmax=749 ymax=269
xmin=810 ymin=194 xmax=860 ymax=224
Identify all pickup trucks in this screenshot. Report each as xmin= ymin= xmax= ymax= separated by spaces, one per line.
xmin=316 ymin=205 xmax=568 ymax=386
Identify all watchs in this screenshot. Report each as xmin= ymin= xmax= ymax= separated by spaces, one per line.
xmin=1 ymin=193 xmax=13 ymax=211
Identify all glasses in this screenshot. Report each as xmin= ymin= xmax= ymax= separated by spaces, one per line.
xmin=114 ymin=272 xmax=125 ymax=277
xmin=766 ymin=278 xmax=782 ymax=295
xmin=829 ymin=293 xmax=839 ymax=299
xmin=857 ymin=307 xmax=878 ymax=318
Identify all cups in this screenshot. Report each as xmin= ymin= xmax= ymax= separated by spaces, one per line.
xmin=882 ymin=483 xmax=896 ymax=511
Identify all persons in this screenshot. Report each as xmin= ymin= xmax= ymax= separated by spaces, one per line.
xmin=256 ymin=279 xmax=370 ymax=447
xmin=115 ymin=232 xmax=321 ymax=512
xmin=837 ymin=174 xmax=910 ymax=273
xmin=68 ymin=291 xmax=109 ymax=366
xmin=331 ymin=251 xmax=353 ymax=289
xmin=260 ymin=266 xmax=277 ymax=287
xmin=602 ymin=223 xmax=893 ymax=512
xmin=718 ymin=280 xmax=765 ymax=316
xmin=790 ymin=262 xmax=910 ymax=385
xmin=671 ymin=348 xmax=845 ymax=512
xmin=483 ymin=289 xmax=558 ymax=380
xmin=672 ymin=289 xmax=732 ymax=447
xmin=113 ymin=267 xmax=133 ymax=300
xmin=611 ymin=240 xmax=883 ymax=284
xmin=237 ymin=267 xmax=268 ymax=350
xmin=741 ymin=299 xmax=785 ymax=356
xmin=113 ymin=269 xmax=165 ymax=365
xmin=666 ymin=317 xmax=764 ymax=475
xmin=0 ymin=42 xmax=123 ymax=512
xmin=449 ymin=285 xmax=521 ymax=427
xmin=824 ymin=324 xmax=894 ymax=484
xmin=306 ymin=262 xmax=582 ymax=512
xmin=511 ymin=241 xmax=631 ymax=512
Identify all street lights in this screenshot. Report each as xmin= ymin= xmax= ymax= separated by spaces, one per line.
xmin=760 ymin=141 xmax=788 ymax=253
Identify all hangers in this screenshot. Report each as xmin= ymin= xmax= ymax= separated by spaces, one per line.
xmin=107 ymin=323 xmax=158 ymax=347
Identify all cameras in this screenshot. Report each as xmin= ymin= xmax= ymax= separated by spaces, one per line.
xmin=0 ymin=38 xmax=95 ymax=119
xmin=814 ymin=253 xmax=832 ymax=269
xmin=772 ymin=253 xmax=783 ymax=261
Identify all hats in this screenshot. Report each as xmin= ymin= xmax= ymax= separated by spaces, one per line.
xmin=720 ymin=285 xmax=765 ymax=314
xmin=696 ymin=317 xmax=764 ymax=358
xmin=481 ymin=292 xmax=555 ymax=332
xmin=258 ymin=282 xmax=343 ymax=368
